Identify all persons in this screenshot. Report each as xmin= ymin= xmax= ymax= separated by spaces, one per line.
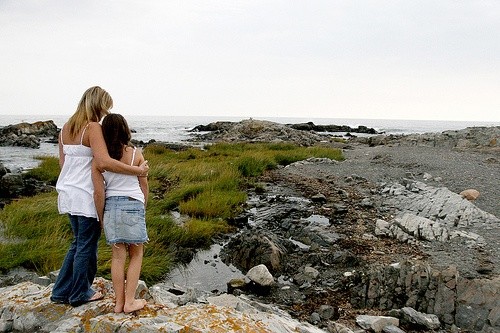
xmin=91 ymin=113 xmax=148 ymax=313
xmin=50 ymin=86 xmax=149 ymax=305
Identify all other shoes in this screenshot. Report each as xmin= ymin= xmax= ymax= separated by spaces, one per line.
xmin=88 ymin=291 xmax=104 ymax=301
xmin=115 ymin=304 xmax=124 ymax=312
xmin=124 ymin=298 xmax=146 ymax=311
